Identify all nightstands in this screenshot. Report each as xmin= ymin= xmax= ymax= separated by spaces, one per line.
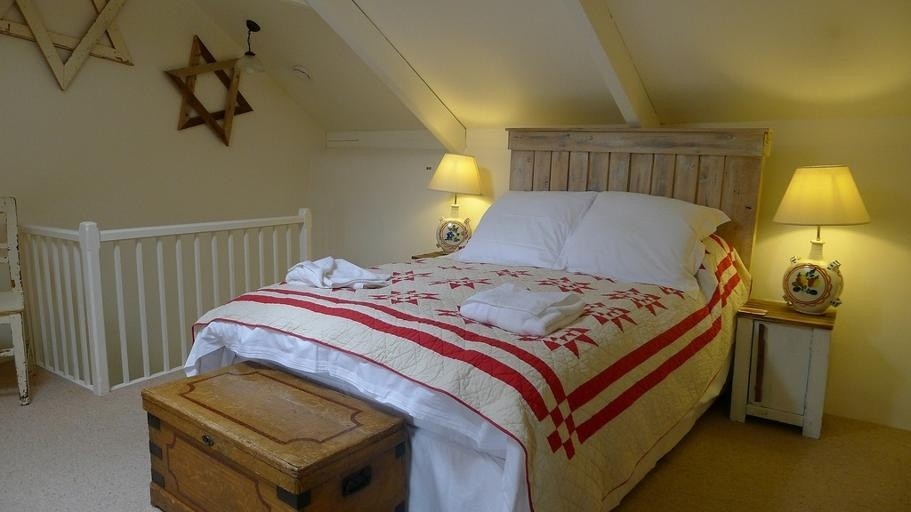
xmin=728 ymin=297 xmax=837 ymax=438
xmin=410 ymin=251 xmax=445 ymax=260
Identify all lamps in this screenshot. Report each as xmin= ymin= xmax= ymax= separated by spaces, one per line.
xmin=424 ymin=152 xmax=484 ymax=254
xmin=235 ymin=19 xmax=263 ymax=75
xmin=772 ymin=164 xmax=872 ymax=317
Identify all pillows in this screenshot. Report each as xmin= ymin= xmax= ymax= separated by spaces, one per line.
xmin=442 ymin=189 xmax=597 ymax=271
xmin=555 ymin=191 xmax=732 ymax=299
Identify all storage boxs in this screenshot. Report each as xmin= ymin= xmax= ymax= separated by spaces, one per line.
xmin=139 ymin=359 xmax=410 ymax=511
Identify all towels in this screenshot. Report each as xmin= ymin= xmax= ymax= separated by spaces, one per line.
xmin=284 ymin=256 xmax=393 ymax=292
xmin=460 ymin=281 xmax=587 ymax=339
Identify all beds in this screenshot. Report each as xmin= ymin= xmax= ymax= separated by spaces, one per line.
xmin=185 ymin=124 xmax=776 ymax=511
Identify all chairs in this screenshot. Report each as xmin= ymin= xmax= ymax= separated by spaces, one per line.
xmin=0 ymin=195 xmax=34 ymax=407
